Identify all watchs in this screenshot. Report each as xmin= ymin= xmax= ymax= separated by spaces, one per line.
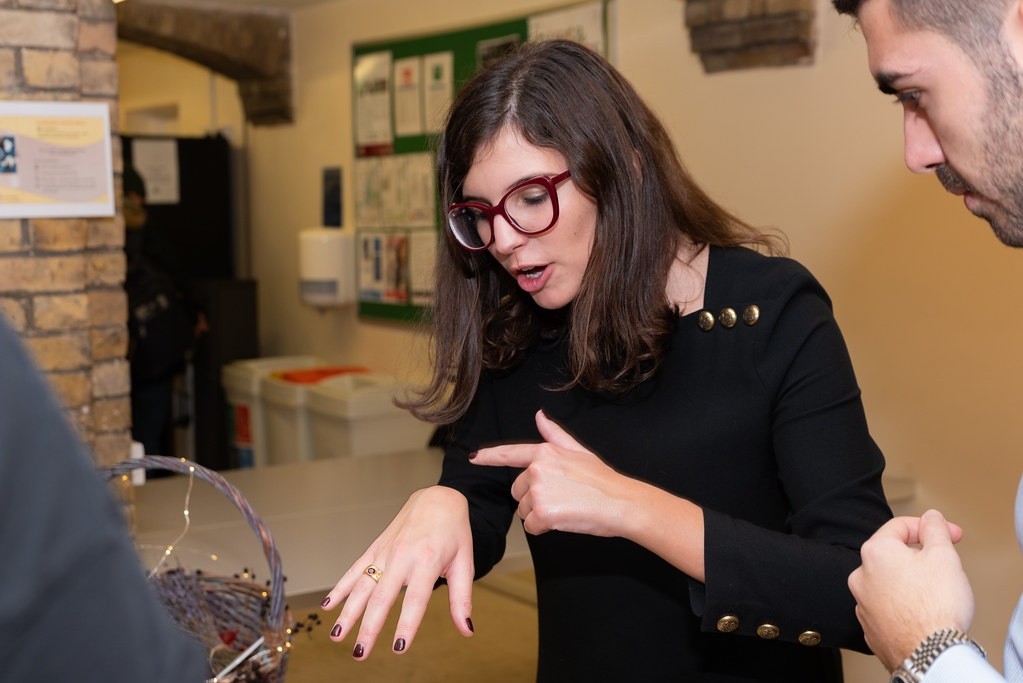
xmin=888 ymin=626 xmax=985 ymax=683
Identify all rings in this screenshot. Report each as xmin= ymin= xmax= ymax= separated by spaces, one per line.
xmin=363 ymin=565 xmax=383 ymax=583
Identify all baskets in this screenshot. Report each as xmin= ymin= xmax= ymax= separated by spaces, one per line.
xmin=100 ymin=455 xmax=291 ymax=682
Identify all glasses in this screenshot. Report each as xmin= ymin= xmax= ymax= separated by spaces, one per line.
xmin=444 ymin=171 xmax=573 ymax=252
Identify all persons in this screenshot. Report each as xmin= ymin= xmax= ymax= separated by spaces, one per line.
xmin=832 ymin=0 xmax=1022 ymax=683
xmin=319 ymin=38 xmax=895 ymax=682
xmin=119 ymin=161 xmax=212 ymax=481
